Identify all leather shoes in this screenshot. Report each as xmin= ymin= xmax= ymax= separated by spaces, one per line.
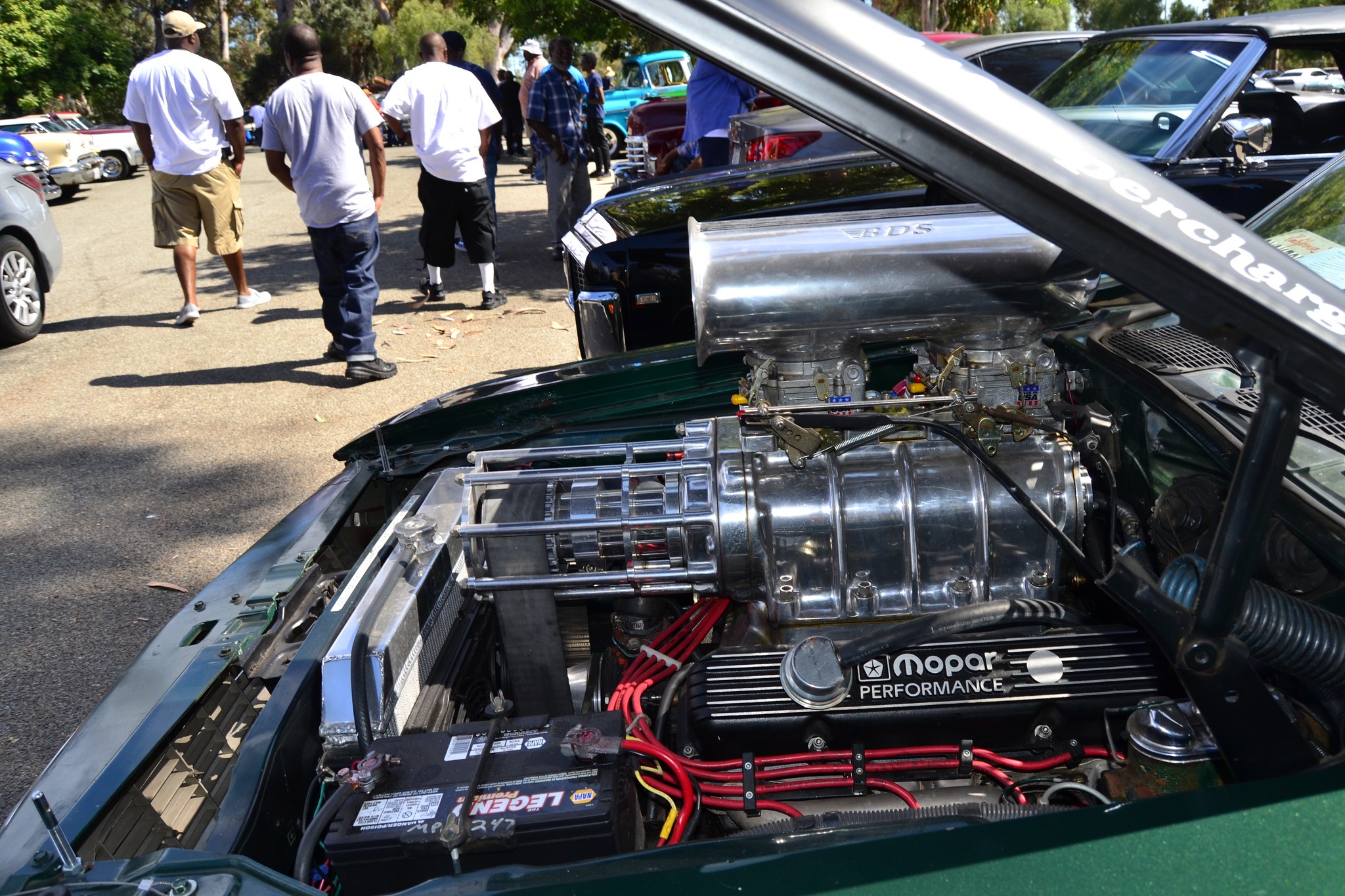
xmin=344 ymin=357 xmax=398 ymax=379
xmin=322 ymin=341 xmax=346 ymax=361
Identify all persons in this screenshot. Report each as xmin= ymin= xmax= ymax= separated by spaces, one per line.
xmin=442 ymin=30 xmax=503 ymax=260
xmin=531 ymin=39 xmax=589 ymax=183
xmin=122 ymin=11 xmax=271 ymax=325
xmin=653 ymin=141 xmax=702 ymax=176
xmin=580 ymin=51 xmax=611 ymax=179
xmin=519 ymin=39 xmax=550 ymax=173
xmin=381 ymin=33 xmax=508 ymax=310
xmin=526 ymin=36 xmax=591 ymax=261
xmin=602 ymin=66 xmax=616 ymax=91
xmin=249 ymin=105 xmax=265 ymax=152
xmin=261 ymin=24 xmax=398 ymax=380
xmin=497 ymin=69 xmax=527 ymax=156
xmin=682 ymin=57 xmax=758 ymax=168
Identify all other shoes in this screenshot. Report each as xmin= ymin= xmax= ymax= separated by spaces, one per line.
xmin=530 ymin=174 xmax=543 ymax=184
xmin=519 ymin=164 xmax=536 ymax=175
xmin=590 ymin=168 xmax=614 ymax=181
xmin=454 ymin=238 xmax=499 ymax=260
xmin=552 ymin=249 xmax=563 ymax=262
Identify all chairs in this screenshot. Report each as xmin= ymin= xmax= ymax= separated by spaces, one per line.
xmin=1239 ymin=92 xmax=1317 ymax=154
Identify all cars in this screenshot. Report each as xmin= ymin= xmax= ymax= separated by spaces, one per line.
xmin=556 ymin=6 xmax=1345 ymax=360
xmin=0 ymin=159 xmax=61 ymax=347
xmin=0 ymin=1 xmax=1345 ymax=895
xmin=0 ymin=128 xmax=63 ymax=201
xmin=15 ymin=111 xmax=154 ymax=182
xmin=360 ymin=77 xmax=412 ymax=148
xmin=579 ymin=30 xmax=1345 ymax=198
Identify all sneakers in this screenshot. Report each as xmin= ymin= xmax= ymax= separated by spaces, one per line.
xmin=235 ymin=288 xmax=271 ymax=309
xmin=176 ymin=303 xmax=200 ymax=324
xmin=481 ymin=289 xmax=508 ymax=310
xmin=418 ymin=276 xmax=445 ymax=301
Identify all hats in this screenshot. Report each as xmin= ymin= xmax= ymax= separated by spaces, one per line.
xmin=519 ymin=39 xmax=543 ymax=55
xmin=603 ymin=66 xmax=616 ymax=77
xmin=161 ymin=10 xmax=206 ymax=38
